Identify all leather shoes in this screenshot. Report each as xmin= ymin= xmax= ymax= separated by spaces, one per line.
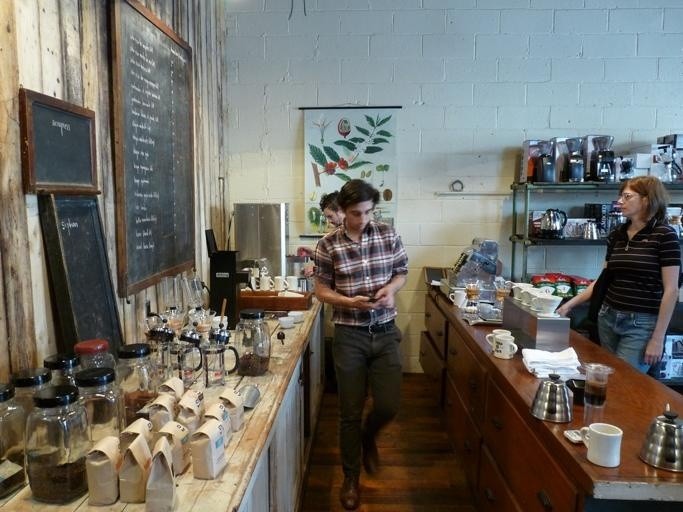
xmin=340 ymin=475 xmax=359 ymax=510
xmin=359 ymin=437 xmax=379 ymax=474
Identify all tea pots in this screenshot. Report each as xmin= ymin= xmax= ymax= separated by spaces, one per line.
xmin=527 ymin=368 xmax=574 ymax=424
xmin=651 ymin=144 xmax=680 ymax=183
xmin=582 ymin=218 xmax=608 ymax=240
xmin=135 ymin=271 xmax=239 ymax=390
xmin=636 ymin=401 xmax=682 ymax=472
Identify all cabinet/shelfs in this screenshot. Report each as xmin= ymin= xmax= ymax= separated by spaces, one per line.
xmin=509 ymin=181 xmax=683 ymax=281
xmin=419 ymin=294 xmax=578 ymax=512
xmin=234 ymin=304 xmax=323 ymax=512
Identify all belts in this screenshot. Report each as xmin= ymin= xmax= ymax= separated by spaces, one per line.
xmin=335 ymin=319 xmax=394 ymax=334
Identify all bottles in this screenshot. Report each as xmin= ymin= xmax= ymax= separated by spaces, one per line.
xmin=0 ymin=337 xmax=156 ymax=508
xmin=231 ymin=308 xmax=271 ymax=376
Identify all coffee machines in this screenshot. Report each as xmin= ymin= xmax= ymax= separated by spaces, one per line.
xmin=533 ymin=136 xmax=617 ymax=184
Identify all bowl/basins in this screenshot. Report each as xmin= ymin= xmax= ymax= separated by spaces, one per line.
xmin=287 ymin=310 xmax=305 ymax=323
xmin=563 ymin=377 xmax=591 ymax=406
xmin=277 ymin=316 xmax=295 ymax=328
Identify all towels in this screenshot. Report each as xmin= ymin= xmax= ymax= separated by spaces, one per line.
xmin=521 ymin=347 xmax=581 ymax=378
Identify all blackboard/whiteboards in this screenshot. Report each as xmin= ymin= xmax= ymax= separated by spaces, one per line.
xmin=37 ymin=192 xmax=126 ymax=369
xmin=111 ymin=0 xmax=195 ymax=296
xmin=19 ymin=87 xmax=103 ymax=197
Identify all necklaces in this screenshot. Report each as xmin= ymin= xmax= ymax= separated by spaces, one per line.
xmin=624 ymin=222 xmax=646 ymax=252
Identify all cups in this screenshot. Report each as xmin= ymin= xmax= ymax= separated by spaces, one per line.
xmin=579 ymin=422 xmax=623 ymax=468
xmin=239 ymin=256 xmax=314 ymax=292
xmin=578 ymin=361 xmax=615 ymax=408
xmin=494 ymin=335 xmax=518 ymax=359
xmin=446 ymin=276 xmax=562 ymax=321
xmin=485 ymin=329 xmax=511 ymax=351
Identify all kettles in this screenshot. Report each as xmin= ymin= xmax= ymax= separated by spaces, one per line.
xmin=538 ymin=208 xmax=567 ymax=240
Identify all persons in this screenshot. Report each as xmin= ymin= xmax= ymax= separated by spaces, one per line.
xmin=298 ymin=191 xmax=348 ymax=281
xmin=556 ymin=177 xmax=679 ymax=379
xmin=315 ymin=177 xmax=407 ymax=509
